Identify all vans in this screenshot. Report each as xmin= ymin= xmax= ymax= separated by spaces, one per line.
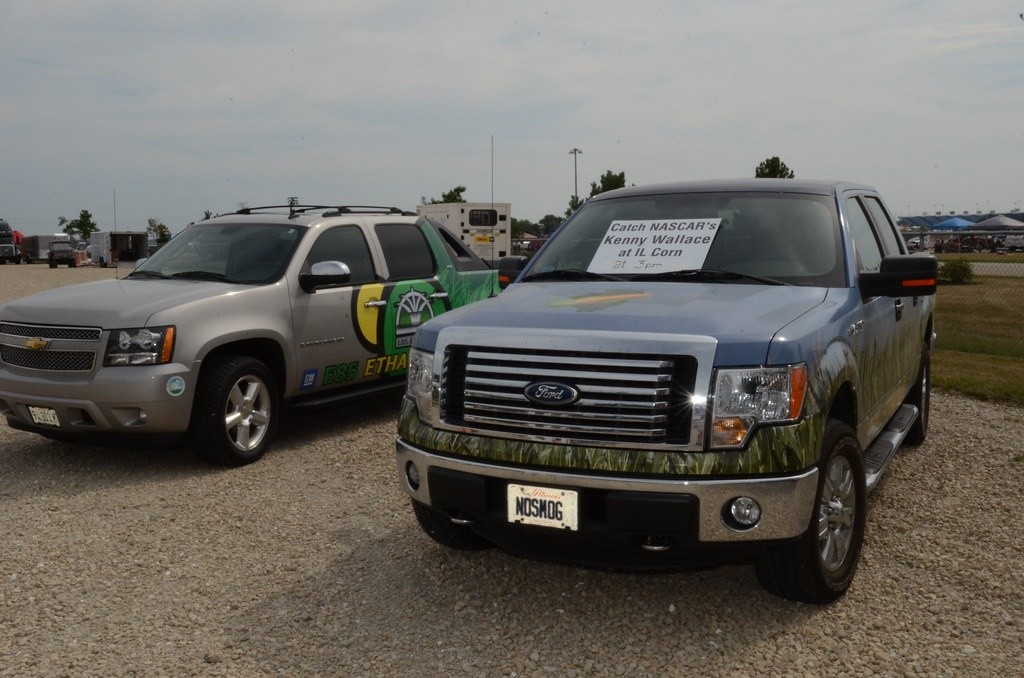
xmin=0 ymin=231 xmax=69 ymax=265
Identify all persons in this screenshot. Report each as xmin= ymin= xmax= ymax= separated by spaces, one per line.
xmin=934 ymin=236 xmax=997 ymax=253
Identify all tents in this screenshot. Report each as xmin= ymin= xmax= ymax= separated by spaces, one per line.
xmin=968 ymin=215 xmax=1024 ymax=240
xmin=931 ymin=215 xmax=976 ymax=253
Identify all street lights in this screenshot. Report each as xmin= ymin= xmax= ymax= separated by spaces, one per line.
xmin=568 ymin=147 xmax=582 ymax=212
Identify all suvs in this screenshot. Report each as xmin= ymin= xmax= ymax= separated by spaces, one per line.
xmin=391 ymin=176 xmax=940 ymax=603
xmin=47 ymin=241 xmax=76 ymax=268
xmin=0 ymin=202 xmax=504 ymax=470
